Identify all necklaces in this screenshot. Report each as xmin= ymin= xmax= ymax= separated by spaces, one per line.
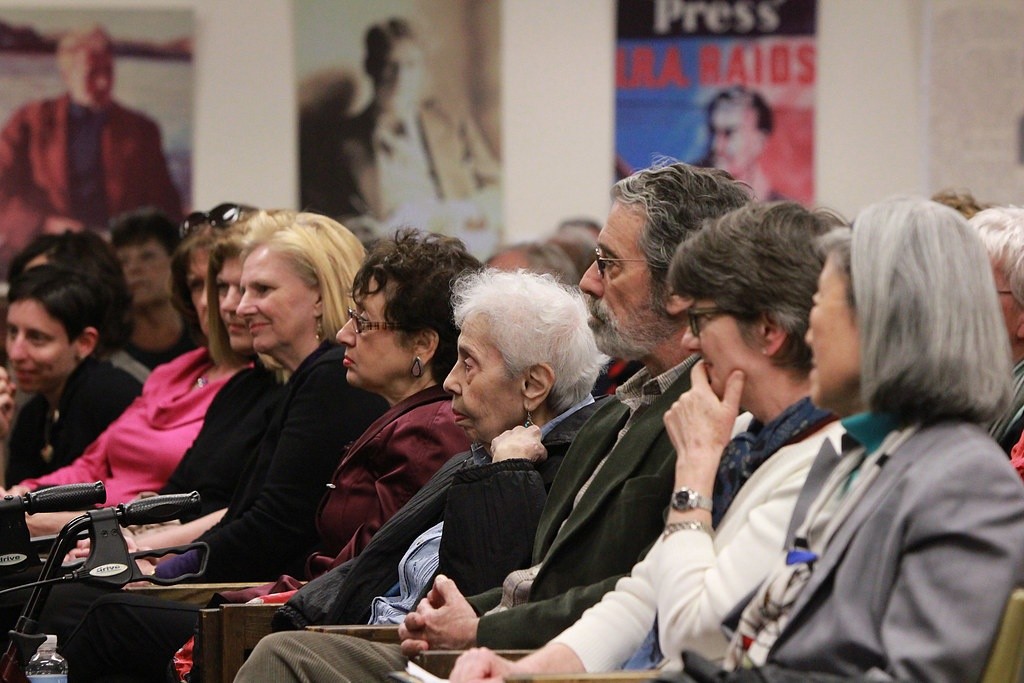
xmin=197 ymin=373 xmax=209 ymax=387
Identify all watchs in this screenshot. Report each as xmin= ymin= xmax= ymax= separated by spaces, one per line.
xmin=672 ymin=488 xmax=714 ymax=512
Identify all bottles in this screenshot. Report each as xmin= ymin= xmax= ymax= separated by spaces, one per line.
xmin=24 ymin=634 xmax=69 ymax=683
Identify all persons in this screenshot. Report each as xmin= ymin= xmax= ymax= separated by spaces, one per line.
xmin=333 ymin=18 xmax=500 ymax=218
xmin=0 ymin=22 xmax=181 ymax=280
xmin=0 ymin=161 xmax=1024 ymax=683
xmin=694 ymin=84 xmax=791 ymax=205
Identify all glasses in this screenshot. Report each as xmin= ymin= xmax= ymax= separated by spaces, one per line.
xmin=179 ymin=202 xmax=257 ymax=242
xmin=688 ymin=304 xmax=758 ymax=337
xmin=346 ymin=307 xmax=415 ymax=334
xmin=596 ymin=247 xmax=660 ymax=279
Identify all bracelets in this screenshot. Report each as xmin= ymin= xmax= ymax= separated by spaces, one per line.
xmin=664 ymin=521 xmax=715 ymax=537
